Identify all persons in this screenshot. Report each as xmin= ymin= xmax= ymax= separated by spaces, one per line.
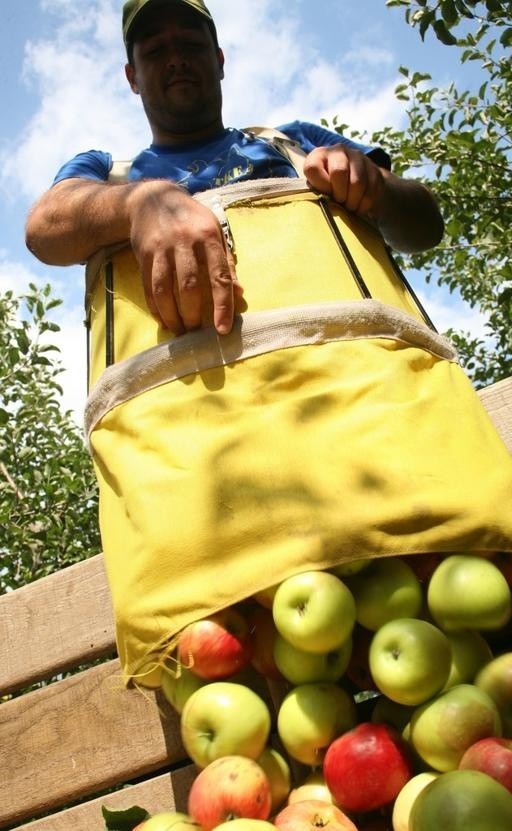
xmin=24 ymin=1 xmax=445 ymax=339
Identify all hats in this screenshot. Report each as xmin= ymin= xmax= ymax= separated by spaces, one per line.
xmin=122 ymin=0 xmax=214 ymax=44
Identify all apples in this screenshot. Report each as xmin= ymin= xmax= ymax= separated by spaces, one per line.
xmin=134 ymin=551 xmax=512 ymax=831
